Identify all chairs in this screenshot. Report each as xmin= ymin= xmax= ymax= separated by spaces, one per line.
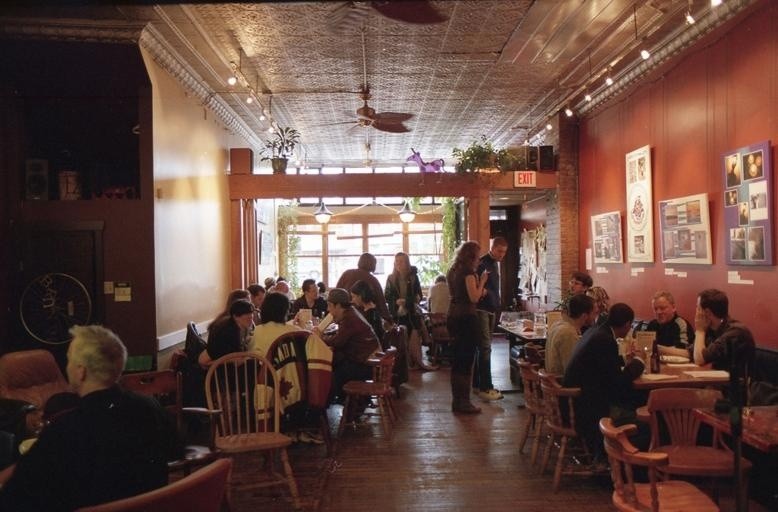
xmin=538 ymin=368 xmax=596 ymax=494
xmin=337 ymin=345 xmax=400 ymax=441
xmin=599 ymin=417 xmax=721 ymax=512
xmin=635 ymin=388 xmax=753 ymax=512
xmin=422 ymin=311 xmax=455 ymax=367
xmin=524 ymin=342 xmax=546 ymax=430
xmin=204 ymin=352 xmax=301 ymax=511
xmin=518 ymin=361 xmax=540 ymax=467
xmin=119 ymin=370 xmax=183 ymax=440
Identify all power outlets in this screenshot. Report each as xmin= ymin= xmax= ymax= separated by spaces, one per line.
xmin=114 ymin=281 xmax=133 ymax=301
xmin=103 ymin=280 xmax=113 ymax=294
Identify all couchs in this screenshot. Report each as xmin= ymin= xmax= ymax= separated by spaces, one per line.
xmin=74 ymin=455 xmax=234 ymax=512
xmin=1 ymin=349 xmax=73 ymax=434
xmin=173 ymin=321 xmax=207 ymax=376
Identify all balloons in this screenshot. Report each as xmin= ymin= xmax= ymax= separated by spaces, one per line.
xmin=405 ymin=147 xmax=450 ymax=186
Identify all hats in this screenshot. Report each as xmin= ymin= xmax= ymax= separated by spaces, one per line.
xmin=229 ymin=299 xmax=255 ymax=315
xmin=327 ymin=288 xmax=356 ymax=309
xmin=253 ymin=292 xmax=291 ymax=315
xmin=265 ymin=277 xmax=275 ymax=287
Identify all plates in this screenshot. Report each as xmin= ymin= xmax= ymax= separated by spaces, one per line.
xmin=657 ymin=355 xmax=690 ymax=364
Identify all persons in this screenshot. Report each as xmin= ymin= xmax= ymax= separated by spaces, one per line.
xmin=336 ymin=253 xmax=398 ymax=333
xmin=225 ymin=289 xmax=256 ymax=349
xmin=385 ymin=252 xmax=423 ymax=370
xmin=644 ymin=292 xmax=695 ymax=351
xmin=245 ymin=284 xmax=265 ymax=324
xmin=264 ymin=277 xmax=275 ymax=290
xmin=658 ymin=289 xmax=758 ymax=414
xmin=277 ymin=276 xmax=296 ymax=304
xmin=351 ymin=279 xmax=390 ymax=353
xmin=289 ymin=279 xmax=328 ymax=319
xmin=445 ymin=241 xmax=491 ymax=414
xmin=317 ymin=281 xmax=325 ymax=293
xmin=198 ymin=300 xmax=254 ymax=369
xmin=298 ymin=287 xmax=382 ymax=443
xmin=425 ymin=275 xmax=451 ymax=354
xmin=0 ymin=324 xmax=177 ymax=512
xmin=559 ymin=302 xmax=653 ymax=484
xmin=545 ymin=295 xmax=595 ymax=385
xmin=567 ymin=272 xmax=593 ymax=296
xmin=585 ymin=287 xmax=610 ymax=329
xmin=473 ymin=236 xmax=508 ymax=401
xmin=269 ymin=281 xmax=289 ymax=294
xmin=248 ymin=292 xmax=305 ymax=367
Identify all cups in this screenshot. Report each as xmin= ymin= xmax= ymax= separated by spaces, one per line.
xmin=534 ymin=311 xmax=547 ymax=338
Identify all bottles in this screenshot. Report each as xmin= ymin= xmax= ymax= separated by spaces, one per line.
xmin=312 ymin=308 xmax=319 ymax=326
xmin=649 ymin=339 xmax=659 ymax=374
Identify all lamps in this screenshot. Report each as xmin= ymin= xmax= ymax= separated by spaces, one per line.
xmin=246 ymin=91 xmax=254 ymax=104
xmin=227 ymin=68 xmax=240 ymax=85
xmin=314 ymin=195 xmax=333 ymax=226
xmin=566 ymin=97 xmax=574 ymax=118
xmin=586 ymin=84 xmax=592 ymax=102
xmin=641 ymin=36 xmax=649 ymax=59
xmin=398 ymin=196 xmax=418 ymax=224
xmin=686 ymin=1 xmax=696 ymax=27
xmin=544 ymin=89 xmax=553 ymax=132
xmin=260 ymin=108 xmax=267 ymax=122
xmin=605 ymin=65 xmax=613 ymax=87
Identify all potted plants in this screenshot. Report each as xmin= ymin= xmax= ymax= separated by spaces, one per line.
xmin=260 ymin=124 xmax=301 ymax=173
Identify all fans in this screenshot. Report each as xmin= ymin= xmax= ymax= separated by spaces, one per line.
xmin=326 ymin=0 xmax=449 ymax=32
xmin=360 ymin=142 xmax=375 ymax=167
xmin=309 ymin=94 xmax=411 ymax=136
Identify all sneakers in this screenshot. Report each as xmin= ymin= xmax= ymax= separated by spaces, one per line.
xmin=298 ymin=431 xmax=325 ymax=443
xmin=284 ymin=431 xmax=298 ymax=442
xmin=472 ymin=387 xmax=480 ymax=393
xmin=478 ymin=389 xmax=504 ymax=401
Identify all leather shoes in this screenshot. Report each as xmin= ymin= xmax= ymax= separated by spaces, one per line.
xmin=452 ymin=404 xmax=481 ymax=415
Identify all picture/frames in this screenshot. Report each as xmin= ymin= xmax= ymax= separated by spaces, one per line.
xmin=625 ymin=144 xmax=654 ymax=263
xmin=719 ymin=139 xmax=775 ymax=268
xmin=658 ymin=193 xmax=710 ymax=265
xmin=260 ymin=229 xmax=274 ymax=265
xmin=589 ymin=211 xmax=624 ymax=263
xmin=257 ymin=200 xmax=269 ymax=227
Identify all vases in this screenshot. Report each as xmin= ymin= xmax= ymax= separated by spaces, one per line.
xmin=56 ymin=171 xmax=86 ymax=199
